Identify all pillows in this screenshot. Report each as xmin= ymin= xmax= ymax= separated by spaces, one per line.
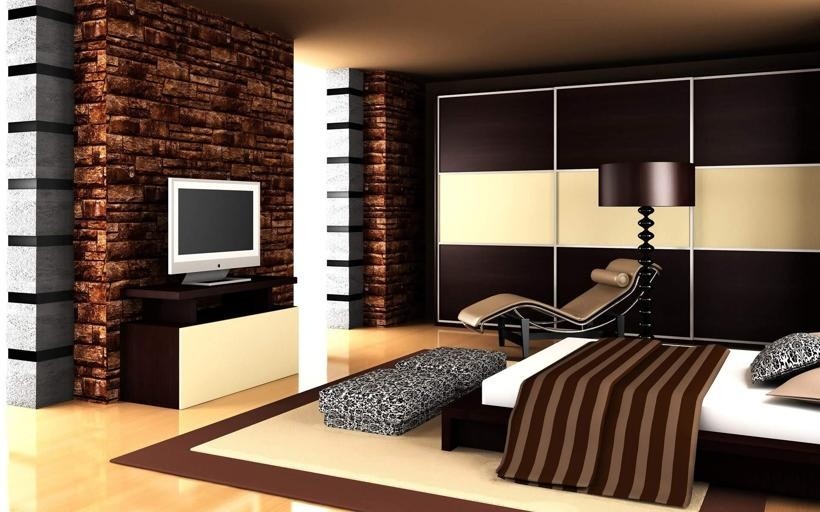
xmin=766 ymin=331 xmax=820 ymax=404
xmin=591 ymin=267 xmax=630 ymax=289
xmin=749 ymin=333 xmax=820 ymax=384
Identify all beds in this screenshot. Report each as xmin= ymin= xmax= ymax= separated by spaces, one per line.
xmin=441 ymin=336 xmax=820 ymax=496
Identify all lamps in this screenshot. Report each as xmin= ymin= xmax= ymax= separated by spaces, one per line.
xmin=598 ymin=158 xmax=695 ymax=338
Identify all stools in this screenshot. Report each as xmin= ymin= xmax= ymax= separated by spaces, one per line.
xmin=396 ymin=347 xmax=507 ymax=398
xmin=318 ymin=368 xmax=458 ymax=437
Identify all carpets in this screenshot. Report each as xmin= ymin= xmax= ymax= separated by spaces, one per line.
xmin=109 ymin=349 xmax=768 ymax=511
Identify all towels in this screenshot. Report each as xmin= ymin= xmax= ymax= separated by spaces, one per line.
xmin=495 ymin=333 xmax=729 ymax=508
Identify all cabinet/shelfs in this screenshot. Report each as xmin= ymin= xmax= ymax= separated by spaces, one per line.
xmin=117 ymin=277 xmax=299 ymax=409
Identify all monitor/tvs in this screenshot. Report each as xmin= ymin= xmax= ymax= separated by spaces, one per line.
xmin=167 ymin=177 xmax=261 ymax=286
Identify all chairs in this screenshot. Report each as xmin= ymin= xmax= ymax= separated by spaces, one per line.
xmin=457 ymin=254 xmax=664 ymax=360
